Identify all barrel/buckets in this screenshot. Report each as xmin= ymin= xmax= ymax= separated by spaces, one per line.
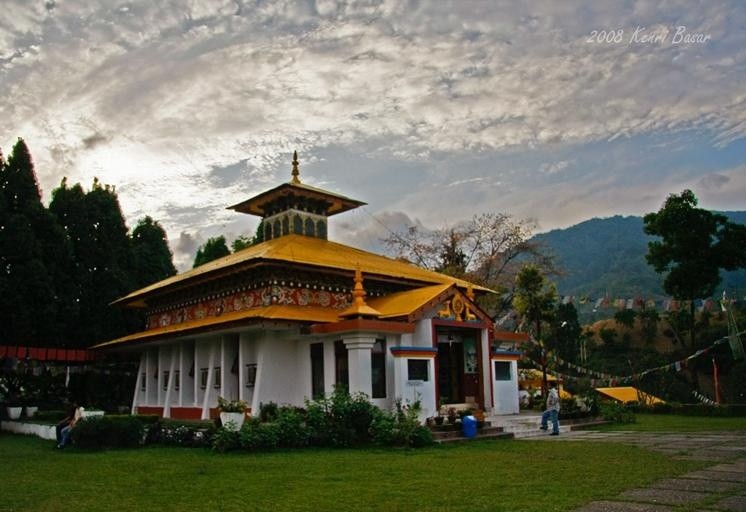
xmin=462 ymin=415 xmax=477 ymax=438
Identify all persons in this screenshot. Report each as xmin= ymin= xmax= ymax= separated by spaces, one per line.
xmin=55 ymin=400 xmax=86 ymax=443
xmin=540 ymin=379 xmax=561 ymax=436
xmin=57 ymin=404 xmax=80 ymax=450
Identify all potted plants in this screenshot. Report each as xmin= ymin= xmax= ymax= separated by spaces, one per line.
xmin=214 ymin=395 xmax=249 ymax=433
xmin=0 ymin=363 xmax=57 ymax=420
xmin=81 ymin=406 xmax=104 ymax=421
xmin=434 ymin=395 xmax=485 ymax=429
